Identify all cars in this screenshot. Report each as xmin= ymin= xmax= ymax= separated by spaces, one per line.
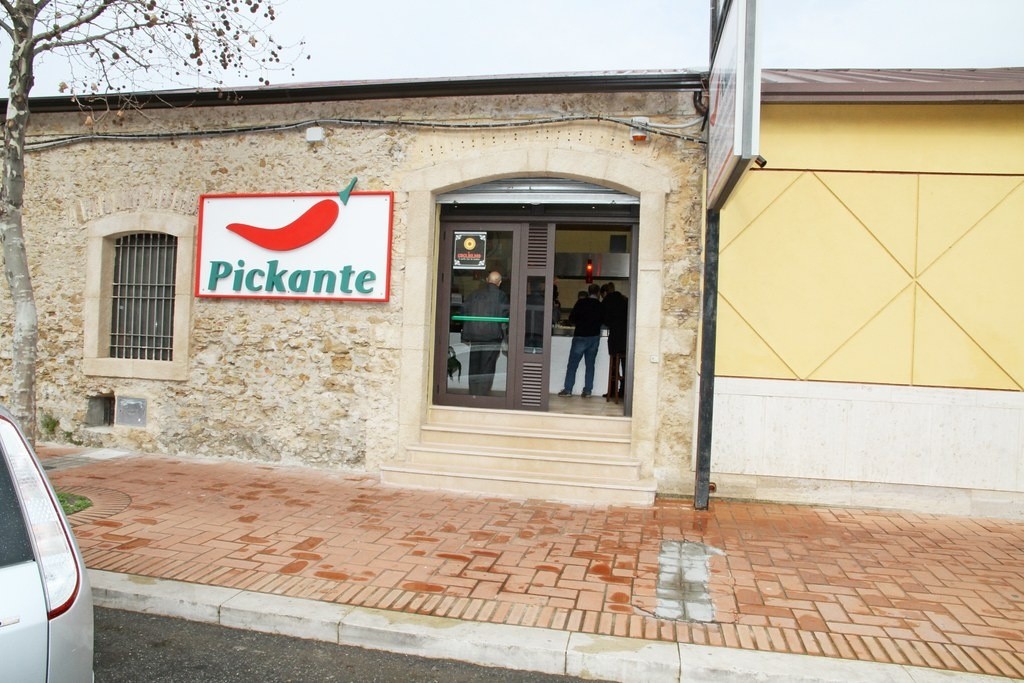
xmin=0 ymin=406 xmax=97 ymax=683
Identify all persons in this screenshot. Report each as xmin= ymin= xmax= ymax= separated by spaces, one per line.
xmin=526 ymin=283 xmax=561 ymax=347
xmin=578 ymin=291 xmax=588 ymax=299
xmin=601 ymin=282 xmax=628 ymax=398
xmin=558 ymin=284 xmax=604 ymax=397
xmin=462 ymin=271 xmax=509 ymax=394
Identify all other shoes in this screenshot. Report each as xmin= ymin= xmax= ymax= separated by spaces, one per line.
xmin=558 ymin=390 xmax=572 ymax=397
xmin=581 ymin=392 xmax=591 ymax=398
xmin=603 ymin=394 xmax=614 ymax=397
